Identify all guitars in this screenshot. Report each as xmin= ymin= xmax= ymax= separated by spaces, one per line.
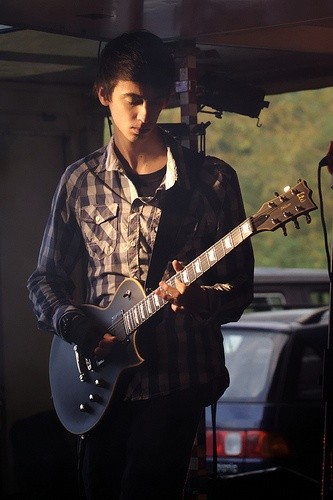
xmin=47 ymin=176 xmax=321 ymax=439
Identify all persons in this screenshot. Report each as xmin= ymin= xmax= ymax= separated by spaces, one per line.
xmin=23 ymin=31 xmax=254 ymax=499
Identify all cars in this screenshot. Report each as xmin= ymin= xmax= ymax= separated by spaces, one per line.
xmin=191 ymin=305 xmax=333 ymax=488
xmin=239 ymin=265 xmax=333 ymax=311
